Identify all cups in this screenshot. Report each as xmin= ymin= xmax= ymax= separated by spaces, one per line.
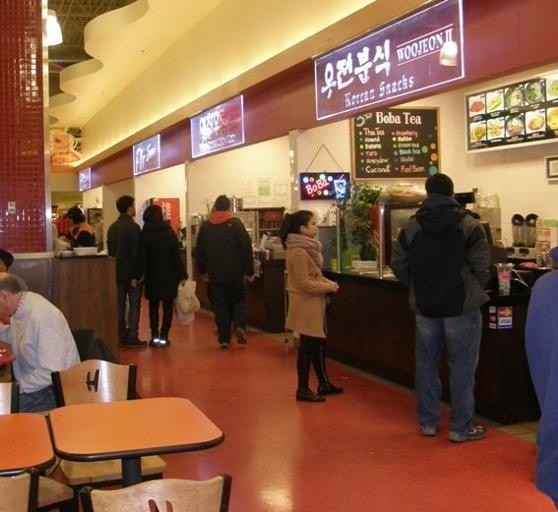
xmin=334 ymin=174 xmax=346 ymax=199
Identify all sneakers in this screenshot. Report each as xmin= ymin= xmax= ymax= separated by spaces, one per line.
xmin=296 ymin=388 xmax=324 ymax=402
xmin=448 ymin=424 xmax=487 ymax=442
xmin=126 ymin=335 xmax=148 ymax=346
xmin=119 ymin=329 xmax=127 ymax=346
xmin=39 ymin=456 xmax=61 ymax=478
xmin=235 ymin=325 xmax=248 ymax=345
xmin=318 ymin=381 xmax=343 ymax=395
xmin=419 ymin=422 xmax=441 ymax=436
xmin=218 ymin=343 xmax=230 ymax=350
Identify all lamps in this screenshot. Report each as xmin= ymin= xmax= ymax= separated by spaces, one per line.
xmin=42 ymin=9 xmax=64 ymax=46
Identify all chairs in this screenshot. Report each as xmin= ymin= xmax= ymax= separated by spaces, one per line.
xmin=50 ymin=359 xmax=166 ymax=495
xmin=0 ymin=466 xmax=40 ymax=512
xmin=0 ymin=380 xmax=75 ymax=512
xmin=78 ymin=473 xmax=231 ymax=512
xmin=73 ymin=329 xmax=103 ymax=361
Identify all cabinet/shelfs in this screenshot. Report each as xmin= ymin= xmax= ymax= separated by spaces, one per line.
xmin=243 ymin=207 xmax=283 ymax=245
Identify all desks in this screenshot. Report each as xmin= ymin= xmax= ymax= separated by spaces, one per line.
xmin=0 ymin=412 xmax=56 ymax=512
xmin=49 ymin=396 xmax=225 ymax=489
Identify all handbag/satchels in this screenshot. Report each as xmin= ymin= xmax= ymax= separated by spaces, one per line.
xmin=177 ymin=280 xmax=200 ymax=312
xmin=173 ymin=297 xmax=195 ymax=325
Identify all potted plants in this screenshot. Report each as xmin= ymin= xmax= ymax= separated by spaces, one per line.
xmin=332 ymin=183 xmax=383 ymax=271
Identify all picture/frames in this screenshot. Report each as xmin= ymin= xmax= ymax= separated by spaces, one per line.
xmin=545 ymin=156 xmax=558 ymax=180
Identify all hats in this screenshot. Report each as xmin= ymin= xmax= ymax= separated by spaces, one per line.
xmin=143 ymin=206 xmax=162 ymax=222
xmin=68 ymin=207 xmax=86 ymax=224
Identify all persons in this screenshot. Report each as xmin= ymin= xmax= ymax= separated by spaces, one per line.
xmin=525 ymin=247 xmax=558 ymax=512
xmin=196 ymin=194 xmax=255 ymax=350
xmin=52 ymin=205 xmax=58 ymax=213
xmin=0 ymin=249 xmax=13 ymax=273
xmin=107 ymin=194 xmax=148 ymax=347
xmin=60 ymin=207 xmax=96 ymax=248
xmin=280 ymin=210 xmax=344 ymax=401
xmin=137 ymin=206 xmax=189 ymax=346
xmin=0 ymin=272 xmax=82 ymax=414
xmin=389 ymin=173 xmax=491 ymax=442
xmin=94 ymin=211 xmax=105 ymax=248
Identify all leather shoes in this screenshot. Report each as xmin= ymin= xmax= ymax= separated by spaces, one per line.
xmin=151 ymin=337 xmax=159 ymax=346
xmin=159 ymin=338 xmax=170 ymax=347
